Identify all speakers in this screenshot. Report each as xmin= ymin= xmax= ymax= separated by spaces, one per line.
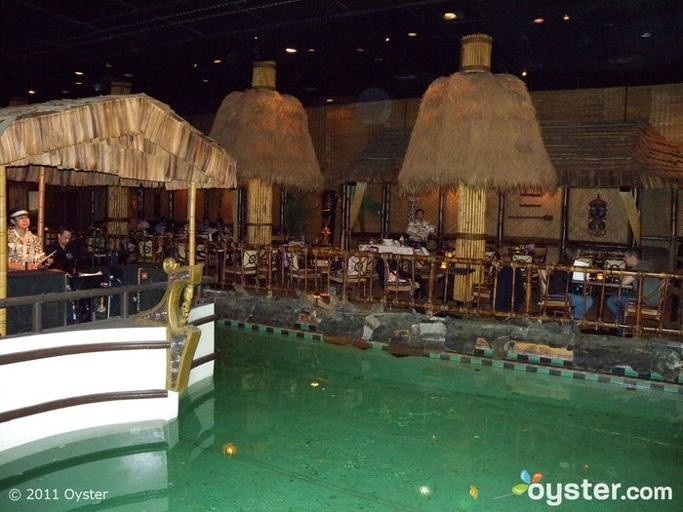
xmin=123 ymin=267 xmax=161 ymax=314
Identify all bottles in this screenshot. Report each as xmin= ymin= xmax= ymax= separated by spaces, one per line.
xmin=399 ymin=234 xmax=404 ymax=247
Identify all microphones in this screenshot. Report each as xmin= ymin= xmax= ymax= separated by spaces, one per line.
xmin=24 ymin=259 xmax=29 ymax=275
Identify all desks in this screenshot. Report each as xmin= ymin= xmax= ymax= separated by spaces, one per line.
xmin=6 ymin=269 xmax=66 ymax=335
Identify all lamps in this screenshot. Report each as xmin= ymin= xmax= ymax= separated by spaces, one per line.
xmin=588 ymin=193 xmax=607 ymax=236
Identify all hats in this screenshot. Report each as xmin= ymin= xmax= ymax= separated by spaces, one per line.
xmin=9 ymin=207 xmax=29 ymax=218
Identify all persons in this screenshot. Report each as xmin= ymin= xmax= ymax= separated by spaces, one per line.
xmin=6 ymin=206 xmax=664 ymax=338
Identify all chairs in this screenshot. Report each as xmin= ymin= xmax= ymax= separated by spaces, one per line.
xmin=71 ymin=230 xmax=683 ymax=338
